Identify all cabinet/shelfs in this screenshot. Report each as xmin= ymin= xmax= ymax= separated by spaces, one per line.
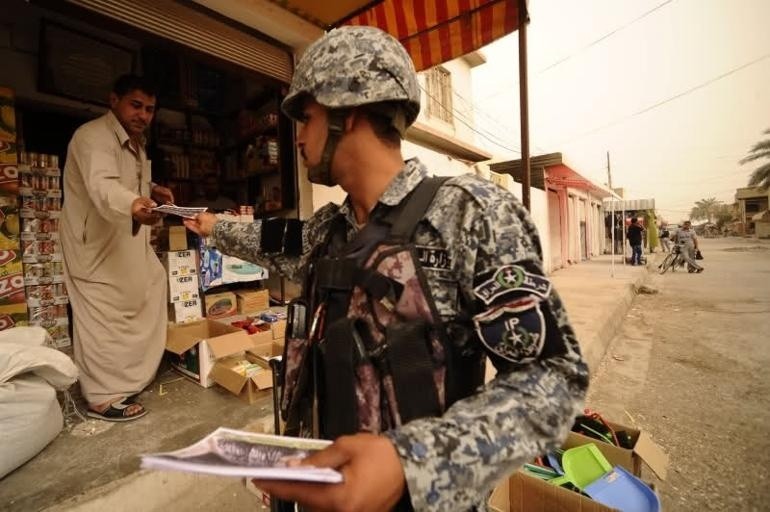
xmin=149 ymin=82 xmax=294 ymax=213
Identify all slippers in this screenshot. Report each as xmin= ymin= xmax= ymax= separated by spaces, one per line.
xmin=87 ymin=397 xmax=149 ymax=422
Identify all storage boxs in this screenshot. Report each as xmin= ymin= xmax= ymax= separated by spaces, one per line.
xmin=18 ymin=152 xmax=304 ymax=403
xmin=485 ymin=411 xmax=669 ymax=512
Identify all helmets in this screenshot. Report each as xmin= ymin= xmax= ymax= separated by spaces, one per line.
xmin=281 ymin=26 xmax=420 ymax=131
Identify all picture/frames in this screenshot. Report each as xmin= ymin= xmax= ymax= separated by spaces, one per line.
xmin=37 ymin=16 xmax=138 ymax=109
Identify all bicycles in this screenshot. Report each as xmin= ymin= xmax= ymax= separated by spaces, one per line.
xmin=658 ymin=243 xmax=684 ymax=275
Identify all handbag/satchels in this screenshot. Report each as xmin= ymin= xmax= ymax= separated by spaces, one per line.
xmin=696 ymin=250 xmax=703 ymax=260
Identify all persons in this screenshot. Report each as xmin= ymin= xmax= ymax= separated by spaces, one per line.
xmin=658 ymin=222 xmax=672 ymax=253
xmin=183 ymin=24 xmax=588 ymax=512
xmin=628 ymin=218 xmax=646 ymax=265
xmin=187 ymin=172 xmax=237 ymax=209
xmin=674 ymin=221 xmax=704 ymax=273
xmin=57 ymin=71 xmax=176 ymax=422
